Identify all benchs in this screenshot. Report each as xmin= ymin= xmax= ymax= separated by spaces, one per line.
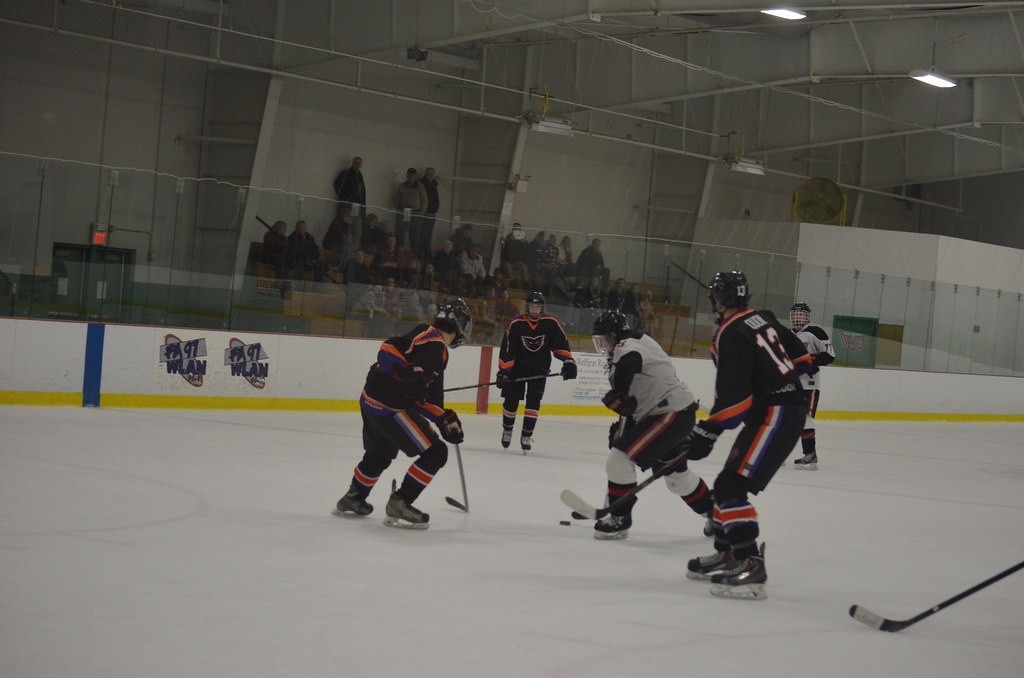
xmin=243 ymin=241 xmax=715 ymax=359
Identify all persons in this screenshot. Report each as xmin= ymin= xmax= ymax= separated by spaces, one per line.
xmin=677 ymin=270 xmax=819 ymax=587
xmin=789 ymin=302 xmax=836 ymax=465
xmin=263 ymin=156 xmax=657 ymax=345
xmin=591 ymin=310 xmax=718 ymax=538
xmin=336 ymin=296 xmax=472 ymax=524
xmin=496 ymin=291 xmax=578 ymax=450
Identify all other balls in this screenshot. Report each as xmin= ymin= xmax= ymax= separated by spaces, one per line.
xmin=559 ymin=521 xmax=571 ymax=526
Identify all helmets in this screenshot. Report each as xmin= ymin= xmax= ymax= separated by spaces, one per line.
xmin=789 ymin=302 xmax=812 ymax=328
xmin=437 ymin=298 xmax=472 ymax=348
xmin=593 ymin=311 xmax=627 ymax=355
xmin=709 ymin=271 xmax=750 ymax=309
xmin=525 ymin=292 xmax=545 ymax=315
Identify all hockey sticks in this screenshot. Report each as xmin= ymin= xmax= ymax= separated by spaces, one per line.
xmin=559 ymin=447 xmax=693 ymax=521
xmin=848 ymin=559 xmax=1024 ymax=632
xmin=446 ymin=444 xmax=468 ymax=512
xmin=671 ymin=261 xmax=709 ymax=290
xmin=571 ymin=413 xmax=626 ymax=520
xmin=443 ymin=369 xmax=561 ymax=392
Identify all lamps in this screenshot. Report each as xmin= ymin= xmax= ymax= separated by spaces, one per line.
xmin=406 ymin=45 xmax=480 ymax=73
xmin=909 ymin=68 xmax=957 ymax=89
xmin=759 ymin=6 xmax=807 ymax=20
xmin=515 ymin=108 xmax=575 ymax=137
xmin=721 ymin=152 xmax=765 ymax=176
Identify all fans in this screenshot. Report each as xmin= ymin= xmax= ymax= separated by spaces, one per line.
xmin=791 ymin=176 xmax=848 ymax=226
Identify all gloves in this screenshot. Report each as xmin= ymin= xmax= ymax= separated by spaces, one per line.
xmin=435 ymin=409 xmax=463 ymax=444
xmin=677 ymin=419 xmax=723 ymax=459
xmin=496 ymin=371 xmax=512 ymax=389
xmin=560 ymin=359 xmax=577 ymax=380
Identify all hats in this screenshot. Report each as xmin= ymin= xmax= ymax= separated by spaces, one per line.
xmin=367 ymin=214 xmax=378 ymax=221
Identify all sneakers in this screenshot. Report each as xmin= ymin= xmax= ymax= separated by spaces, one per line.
xmin=794 ymin=451 xmax=819 ymax=469
xmin=710 ymin=542 xmax=769 ymax=600
xmin=702 ymin=513 xmax=716 ymax=535
xmin=687 ymin=543 xmax=734 ymax=582
xmin=383 ymin=479 xmax=429 ymax=530
xmin=520 ymin=430 xmax=533 ymax=456
xmin=501 ymin=430 xmax=512 ymax=451
xmin=332 ymin=485 xmax=374 ymax=519
xmin=595 ymin=513 xmax=632 ymax=538
